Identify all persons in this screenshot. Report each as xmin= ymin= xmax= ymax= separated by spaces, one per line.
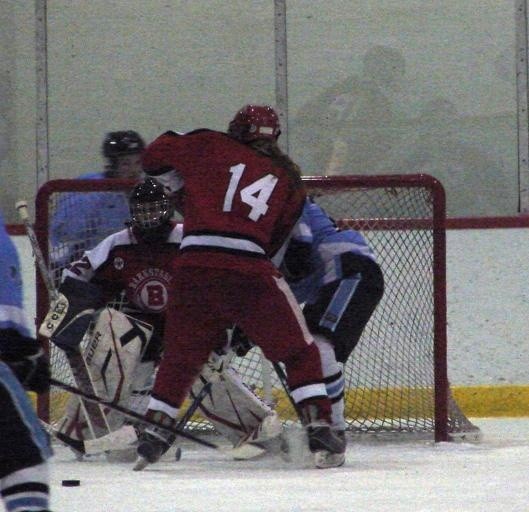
xmin=138 ymin=102 xmax=346 ymax=463
xmin=49 ymin=129 xmax=146 ymax=313
xmin=55 ymin=178 xmax=285 ymax=459
xmin=231 ymin=196 xmax=385 ymax=443
xmin=0 ymin=218 xmax=52 ymax=512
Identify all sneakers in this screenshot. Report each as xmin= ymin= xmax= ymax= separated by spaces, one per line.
xmin=137 ymin=432 xmax=165 ymax=462
xmin=307 ymin=426 xmax=346 ymax=454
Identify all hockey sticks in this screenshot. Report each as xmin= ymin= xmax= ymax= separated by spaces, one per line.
xmin=14 ymin=201 xmax=139 ymax=465
xmin=42 ymin=374 xmax=264 ymax=460
xmin=136 ymin=349 xmax=237 ymax=471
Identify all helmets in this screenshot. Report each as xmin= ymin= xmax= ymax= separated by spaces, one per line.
xmin=226 ymin=104 xmax=281 ymax=143
xmin=129 ymin=178 xmax=174 ymax=232
xmin=104 ymin=130 xmax=144 ymax=167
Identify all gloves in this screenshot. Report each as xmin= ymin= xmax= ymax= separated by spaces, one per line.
xmin=214 ymin=325 xmax=256 ymax=356
xmin=0 ymin=328 xmax=51 ymax=394
